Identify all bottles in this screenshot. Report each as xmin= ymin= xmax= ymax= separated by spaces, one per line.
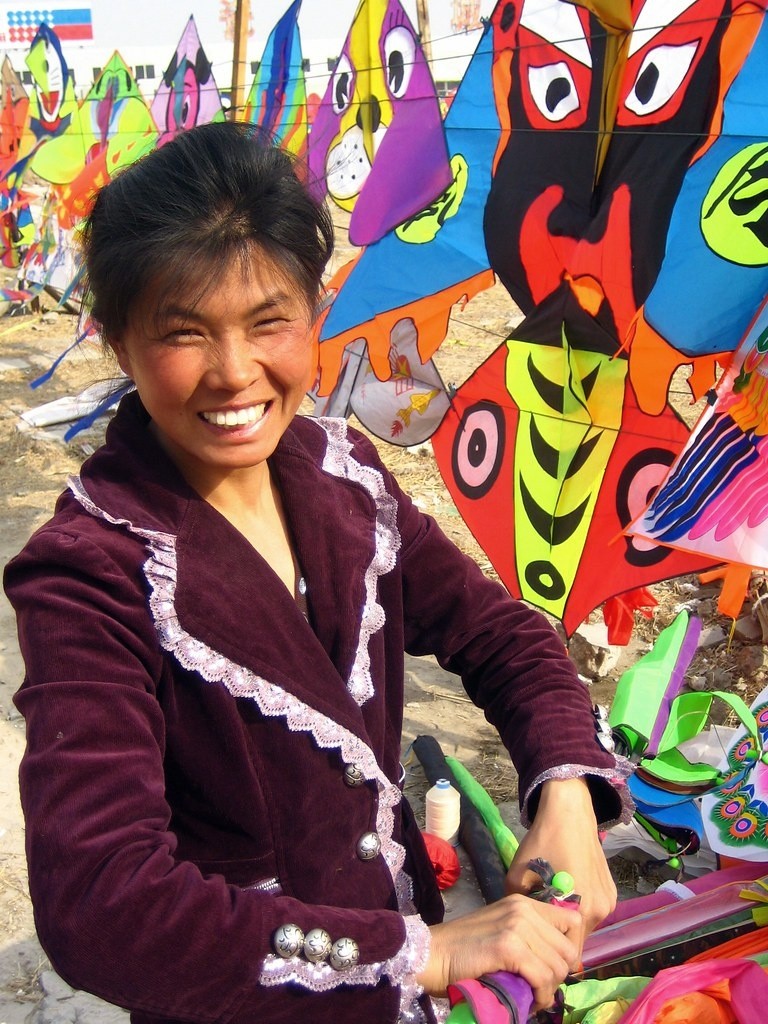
xmin=425 ymin=778 xmax=462 ymax=848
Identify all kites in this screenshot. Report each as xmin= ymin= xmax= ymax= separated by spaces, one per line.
xmin=0 ymin=0 xmax=768 ymax=1024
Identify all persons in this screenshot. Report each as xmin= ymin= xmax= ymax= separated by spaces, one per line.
xmin=1 ymin=121 xmax=635 ymax=1024
